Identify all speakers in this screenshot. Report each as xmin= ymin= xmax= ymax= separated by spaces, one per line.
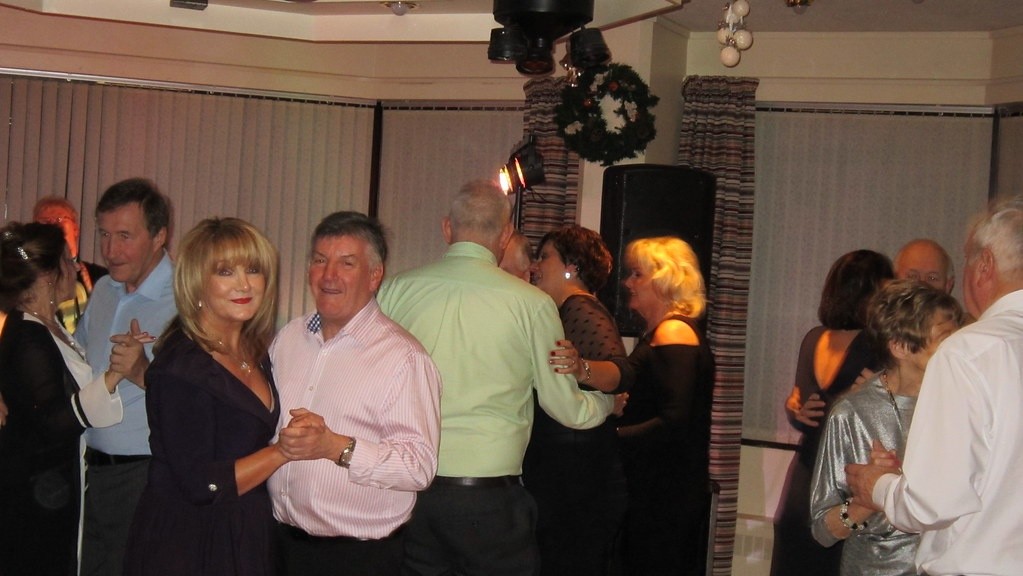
xmin=596 ymin=164 xmax=716 ymax=338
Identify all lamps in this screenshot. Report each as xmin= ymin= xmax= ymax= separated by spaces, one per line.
xmin=382 ymin=0 xmax=418 ymax=16
xmin=787 ymin=0 xmax=811 ymax=16
xmin=499 ymin=143 xmax=546 ymax=197
xmin=486 ymin=0 xmax=612 ymax=77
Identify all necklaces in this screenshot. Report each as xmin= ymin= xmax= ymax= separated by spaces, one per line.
xmin=218 ymin=341 xmax=251 ymax=373
xmin=24 ymin=303 xmax=54 ymax=322
xmin=884 ymin=371 xmax=902 ymax=419
xmin=562 ymin=288 xmax=585 ymax=302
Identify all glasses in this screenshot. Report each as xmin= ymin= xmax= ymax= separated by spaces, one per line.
xmin=43 ymin=216 xmax=75 ymax=226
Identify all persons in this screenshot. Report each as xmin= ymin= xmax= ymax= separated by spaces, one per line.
xmin=268 ymin=211 xmax=445 ymax=576
xmin=522 ymin=224 xmax=631 ymax=576
xmin=0 ymin=222 xmax=157 ymax=576
xmin=73 ymin=180 xmax=177 ymax=576
xmin=33 ymin=198 xmax=109 ymax=338
xmin=377 ymin=177 xmax=630 ymax=576
xmin=623 ymin=237 xmax=714 ymax=575
xmin=770 ymin=239 xmax=977 ymax=576
xmin=498 ymin=234 xmax=532 ymax=282
xmin=844 ymin=207 xmax=1023 ymax=576
xmin=143 ymin=216 xmax=325 ymax=576
xmin=809 ymin=279 xmax=961 ymax=575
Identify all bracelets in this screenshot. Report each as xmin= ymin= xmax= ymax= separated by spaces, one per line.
xmin=841 ymin=497 xmax=868 ymax=531
xmin=581 ymin=358 xmax=591 ymax=383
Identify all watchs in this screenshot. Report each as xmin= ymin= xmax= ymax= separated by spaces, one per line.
xmin=336 ymin=436 xmax=356 ymax=467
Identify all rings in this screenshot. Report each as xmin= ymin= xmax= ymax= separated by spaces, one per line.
xmin=573 ymin=361 xmax=575 ymax=364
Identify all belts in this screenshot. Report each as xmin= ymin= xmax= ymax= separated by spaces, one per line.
xmin=84 ymin=446 xmax=150 ymax=466
xmin=431 ymin=475 xmax=519 ymax=488
